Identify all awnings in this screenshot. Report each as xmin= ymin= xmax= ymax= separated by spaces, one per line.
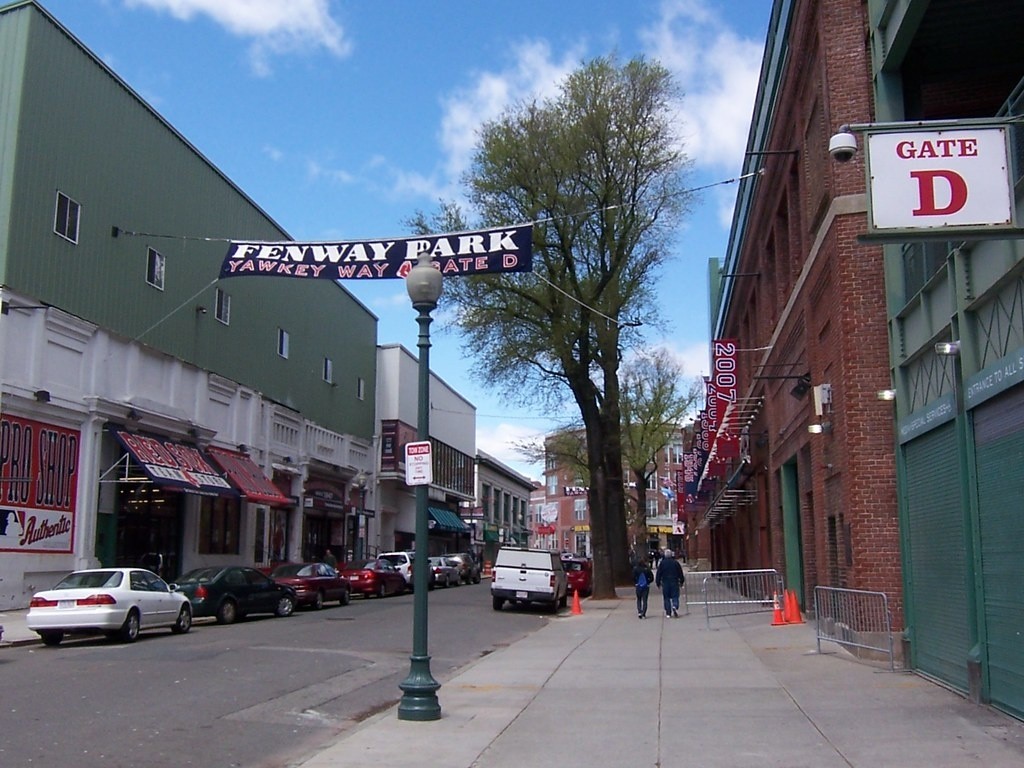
xmin=98 ymin=424 xmax=240 ymax=499
xmin=428 ymin=508 xmax=466 ymax=533
xmin=205 ymin=444 xmax=290 ymax=506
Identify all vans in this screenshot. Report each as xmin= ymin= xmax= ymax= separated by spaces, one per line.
xmin=491 ymin=546 xmax=570 ymax=614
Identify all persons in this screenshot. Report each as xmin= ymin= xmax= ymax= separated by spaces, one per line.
xmin=655 ymin=549 xmax=685 ymax=618
xmin=648 ymin=548 xmax=687 ymax=569
xmin=323 ymin=549 xmax=337 ymax=569
xmin=631 ymin=555 xmax=654 ymax=619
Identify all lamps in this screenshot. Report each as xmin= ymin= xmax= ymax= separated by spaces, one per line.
xmin=34 ymin=390 xmax=50 ymax=402
xmin=237 ymin=444 xmax=249 ymax=453
xmin=284 ymin=456 xmax=293 ymax=463
xmin=128 ymin=411 xmax=143 ymax=422
xmin=189 ymin=429 xmax=199 ymax=437
xmin=790 ymin=377 xmax=811 ymax=400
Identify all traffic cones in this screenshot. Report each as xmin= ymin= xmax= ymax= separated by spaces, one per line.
xmin=770 ymin=587 xmax=808 ymax=625
xmin=572 ymin=589 xmax=585 ymax=615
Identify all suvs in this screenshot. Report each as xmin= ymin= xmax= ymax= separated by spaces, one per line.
xmin=376 ymin=549 xmax=436 ymax=591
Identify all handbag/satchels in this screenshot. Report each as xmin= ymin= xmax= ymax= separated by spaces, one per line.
xmin=637 ymin=573 xmax=648 ymax=590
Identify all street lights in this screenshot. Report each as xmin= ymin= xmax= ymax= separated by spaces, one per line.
xmin=395 ymin=250 xmax=444 ymax=721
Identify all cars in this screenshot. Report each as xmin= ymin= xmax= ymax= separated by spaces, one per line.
xmin=560 ymin=555 xmax=593 ymax=596
xmin=339 ymin=558 xmax=406 ymax=598
xmin=26 ymin=567 xmax=194 ymax=644
xmin=428 ymin=552 xmax=481 ymax=588
xmin=268 ymin=562 xmax=353 ymax=610
xmin=171 ymin=565 xmax=298 ymax=624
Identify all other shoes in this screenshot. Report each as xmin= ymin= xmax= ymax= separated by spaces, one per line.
xmin=642 ymin=614 xmax=646 ymax=619
xmin=666 ymin=614 xmax=670 ymax=618
xmin=672 ymin=606 xmax=678 ymax=617
xmin=638 ymin=612 xmax=641 ymax=618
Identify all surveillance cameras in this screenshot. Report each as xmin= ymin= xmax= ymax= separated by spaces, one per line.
xmin=814 ymin=385 xmax=823 ymax=416
xmin=828 ymin=133 xmax=858 ymax=164
